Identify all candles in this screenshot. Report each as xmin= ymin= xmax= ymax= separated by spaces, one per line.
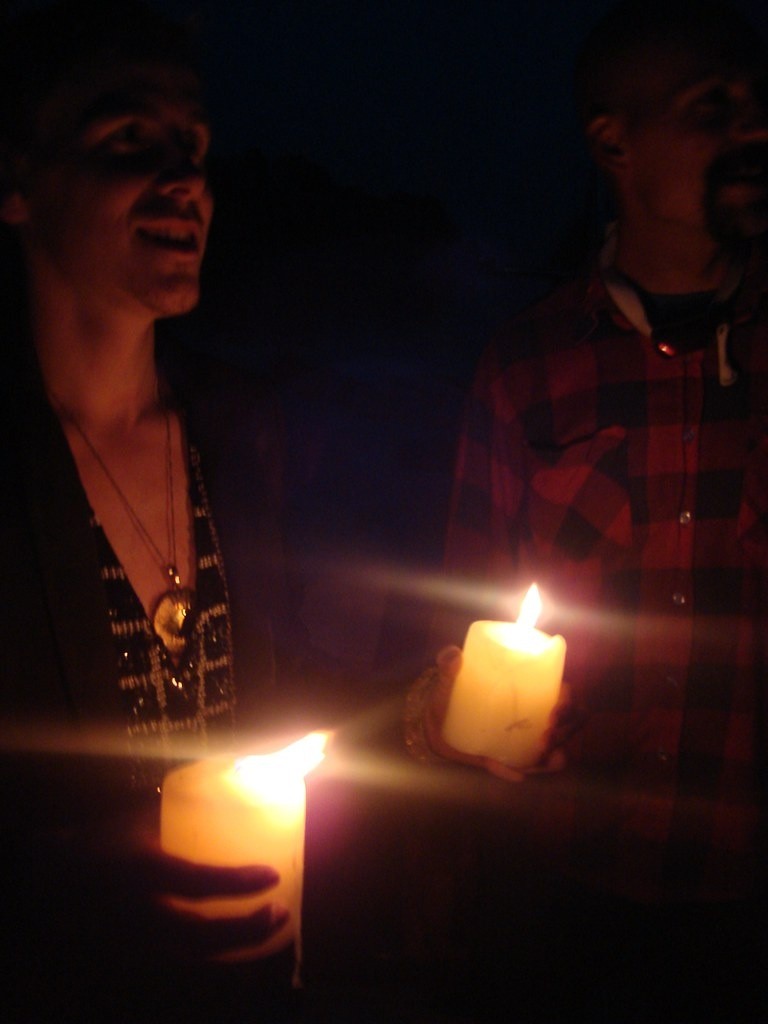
xmin=158 ymin=728 xmax=328 ymax=990
xmin=442 ymin=578 xmax=566 ymax=770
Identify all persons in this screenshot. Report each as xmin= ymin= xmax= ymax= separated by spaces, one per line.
xmin=445 ymin=0 xmax=767 ymax=690
xmin=0 ymin=77 xmax=574 ymax=1021
xmin=1 ymin=1 xmax=299 ymax=1024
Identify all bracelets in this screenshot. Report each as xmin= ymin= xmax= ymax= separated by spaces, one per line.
xmin=404 ymin=658 xmax=444 ymax=767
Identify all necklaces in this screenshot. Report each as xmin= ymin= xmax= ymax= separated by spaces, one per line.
xmin=48 ymin=377 xmax=195 ymax=664
